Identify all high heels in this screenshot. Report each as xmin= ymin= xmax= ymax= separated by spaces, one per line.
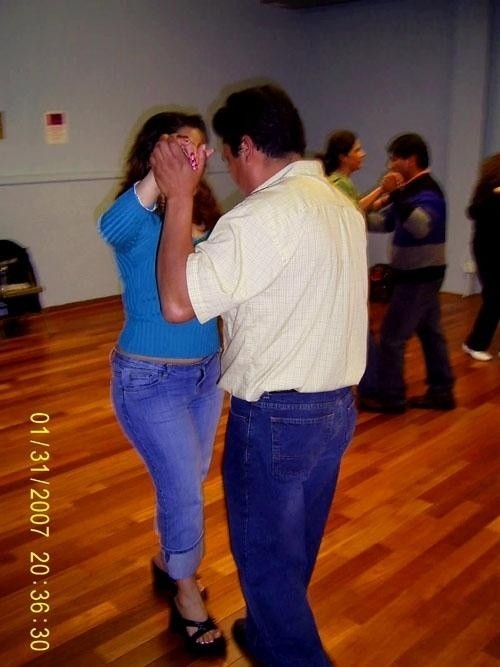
xmin=169 ymin=598 xmax=226 ymax=655
xmin=151 ymin=555 xmax=208 ymax=598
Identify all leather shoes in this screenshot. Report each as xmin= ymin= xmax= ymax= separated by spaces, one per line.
xmin=461 ymin=343 xmax=493 ymax=362
xmin=359 ymin=397 xmax=408 ymax=412
xmin=410 ymin=395 xmax=456 ymax=411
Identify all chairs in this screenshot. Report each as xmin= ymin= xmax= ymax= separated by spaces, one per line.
xmin=0 ymin=240 xmax=42 ymax=337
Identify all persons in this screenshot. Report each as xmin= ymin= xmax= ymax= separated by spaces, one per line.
xmin=149 ymin=83 xmax=370 ymax=667
xmin=97 ymin=112 xmax=229 ymax=658
xmin=462 ymin=153 xmax=500 ymax=363
xmin=359 ymin=133 xmax=456 ymax=415
xmin=317 ymin=127 xmax=401 ymax=400
